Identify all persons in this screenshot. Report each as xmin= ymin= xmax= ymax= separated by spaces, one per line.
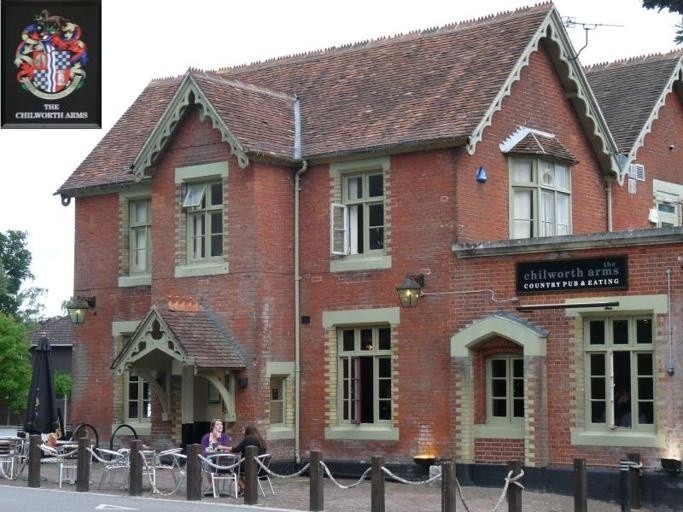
xmin=201 ymin=419 xmax=231 ymax=494
xmin=218 ymin=424 xmax=269 ymax=497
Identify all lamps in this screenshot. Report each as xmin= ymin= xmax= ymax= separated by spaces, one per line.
xmin=414 ymin=454 xmax=441 ymax=468
xmin=660 ymin=456 xmax=683 ymax=472
xmin=395 ymin=271 xmax=424 ymax=309
xmin=65 ymin=295 xmax=95 ymax=325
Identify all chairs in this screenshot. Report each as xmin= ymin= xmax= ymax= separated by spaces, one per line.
xmin=0 ymin=436 xmax=275 ymax=499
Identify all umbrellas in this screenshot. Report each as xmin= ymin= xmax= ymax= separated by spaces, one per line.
xmin=22 ymin=330 xmax=60 ymax=434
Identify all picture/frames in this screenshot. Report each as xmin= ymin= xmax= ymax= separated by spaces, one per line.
xmin=207 ymin=377 xmax=221 ymax=403
